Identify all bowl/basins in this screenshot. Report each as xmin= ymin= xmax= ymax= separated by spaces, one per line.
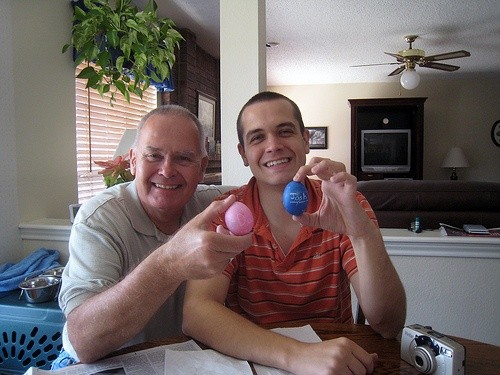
xmin=18 ymin=277 xmax=61 ymax=303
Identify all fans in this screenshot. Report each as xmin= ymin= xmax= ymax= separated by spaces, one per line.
xmin=349 ymin=34 xmax=470 ymax=76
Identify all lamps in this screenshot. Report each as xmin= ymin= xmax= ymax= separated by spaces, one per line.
xmin=400 ymin=62 xmax=421 ymax=90
xmin=440 ymin=147 xmax=471 ymax=181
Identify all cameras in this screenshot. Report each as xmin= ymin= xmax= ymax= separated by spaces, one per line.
xmin=401 ymin=324 xmax=466 ymax=375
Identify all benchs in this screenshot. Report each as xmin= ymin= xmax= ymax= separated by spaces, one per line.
xmin=355 ymin=180 xmax=500 ymax=231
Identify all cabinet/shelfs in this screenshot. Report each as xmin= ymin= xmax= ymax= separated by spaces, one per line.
xmin=347 ymin=97 xmax=428 ymax=181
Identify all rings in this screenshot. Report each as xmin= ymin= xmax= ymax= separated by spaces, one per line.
xmin=229 ymin=256 xmax=233 ymax=262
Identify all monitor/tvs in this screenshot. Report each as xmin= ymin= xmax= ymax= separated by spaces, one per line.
xmin=360 ymin=129 xmax=411 ymax=173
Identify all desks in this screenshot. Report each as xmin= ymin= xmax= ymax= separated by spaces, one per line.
xmin=349 ymin=228 xmax=500 ymax=348
xmin=71 ymin=322 xmax=500 ymax=375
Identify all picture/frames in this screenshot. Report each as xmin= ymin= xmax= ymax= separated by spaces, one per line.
xmin=304 ymin=127 xmax=327 ymax=149
xmin=196 ymin=90 xmax=217 ymax=146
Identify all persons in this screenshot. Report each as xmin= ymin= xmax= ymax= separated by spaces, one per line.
xmin=48 ymin=105 xmax=255 ymax=370
xmin=180 ymin=90 xmax=407 ymax=375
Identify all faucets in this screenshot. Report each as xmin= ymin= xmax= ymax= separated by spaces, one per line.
xmin=408 ymin=217 xmax=423 ymax=233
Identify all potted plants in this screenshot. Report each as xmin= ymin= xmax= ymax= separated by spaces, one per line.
xmin=61 ymin=0 xmax=186 ymax=107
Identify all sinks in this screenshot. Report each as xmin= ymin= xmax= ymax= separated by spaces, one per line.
xmin=379 ymin=228 xmax=443 ymax=237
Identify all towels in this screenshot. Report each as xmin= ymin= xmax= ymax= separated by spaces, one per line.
xmin=0 ymin=248 xmax=62 ymax=299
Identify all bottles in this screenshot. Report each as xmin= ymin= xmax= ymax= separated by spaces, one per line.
xmin=216 ymin=141 xmax=220 ymax=153
xmin=415 ymin=217 xmax=419 ymax=231
